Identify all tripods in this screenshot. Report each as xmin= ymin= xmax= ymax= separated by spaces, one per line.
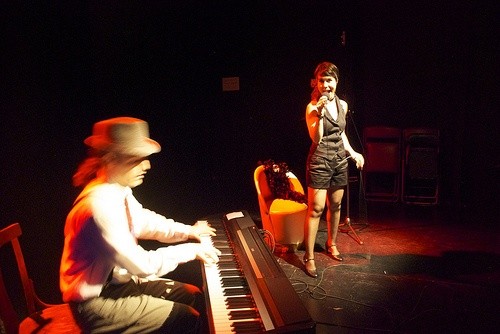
xmin=318 ymin=118 xmax=369 ymax=244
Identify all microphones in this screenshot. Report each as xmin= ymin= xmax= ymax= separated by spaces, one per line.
xmin=323 ymin=92 xmax=329 ymax=105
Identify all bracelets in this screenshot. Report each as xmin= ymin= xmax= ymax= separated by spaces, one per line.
xmin=316 ymin=114 xmax=324 ymax=119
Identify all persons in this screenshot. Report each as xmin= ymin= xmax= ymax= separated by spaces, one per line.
xmin=59 ymin=117 xmax=222 ymax=334
xmin=303 ymin=62 xmax=365 ymax=278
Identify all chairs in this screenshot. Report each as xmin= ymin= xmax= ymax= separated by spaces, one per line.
xmin=0 ymin=223 xmax=83 ymax=334
xmin=362 ymin=125 xmax=440 ymax=206
xmin=254 ymin=165 xmax=308 ymax=254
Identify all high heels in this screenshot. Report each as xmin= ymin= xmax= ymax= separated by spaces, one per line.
xmin=303 ymin=254 xmax=318 ymax=277
xmin=325 ymin=241 xmax=344 ymax=262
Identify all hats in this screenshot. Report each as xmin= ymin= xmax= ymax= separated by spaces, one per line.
xmin=84 ymin=117 xmax=161 ymax=159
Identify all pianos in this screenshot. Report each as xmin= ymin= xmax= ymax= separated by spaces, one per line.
xmin=197 ymin=208 xmax=316 ymax=334
